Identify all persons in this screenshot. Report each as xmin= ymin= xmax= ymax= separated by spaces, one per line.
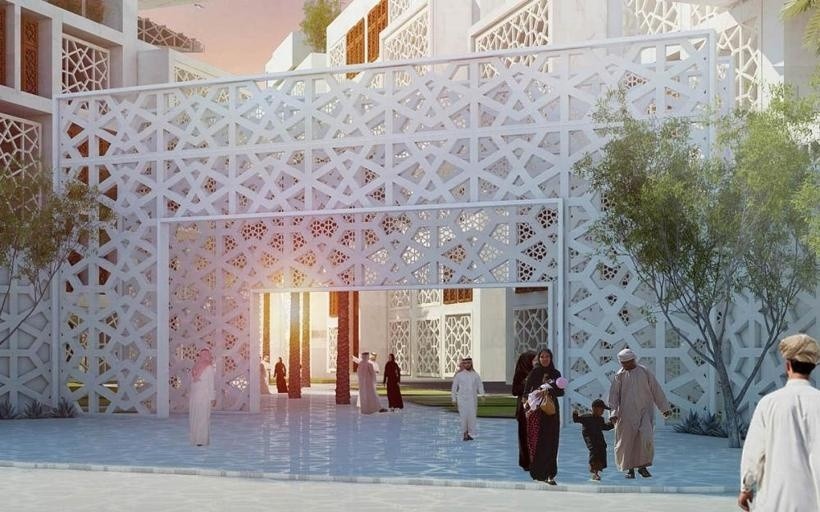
xmin=188 ymin=349 xmax=217 ymax=445
xmin=608 ymin=349 xmax=673 ymax=478
xmin=259 ymin=355 xmax=288 ymax=394
xmin=353 ymin=351 xmax=403 ymax=414
xmin=573 ymin=399 xmax=616 ymax=480
xmin=451 ymin=357 xmax=485 ymax=441
xmin=512 ymin=348 xmax=567 ymax=486
xmin=738 ymin=333 xmax=820 ymax=512
